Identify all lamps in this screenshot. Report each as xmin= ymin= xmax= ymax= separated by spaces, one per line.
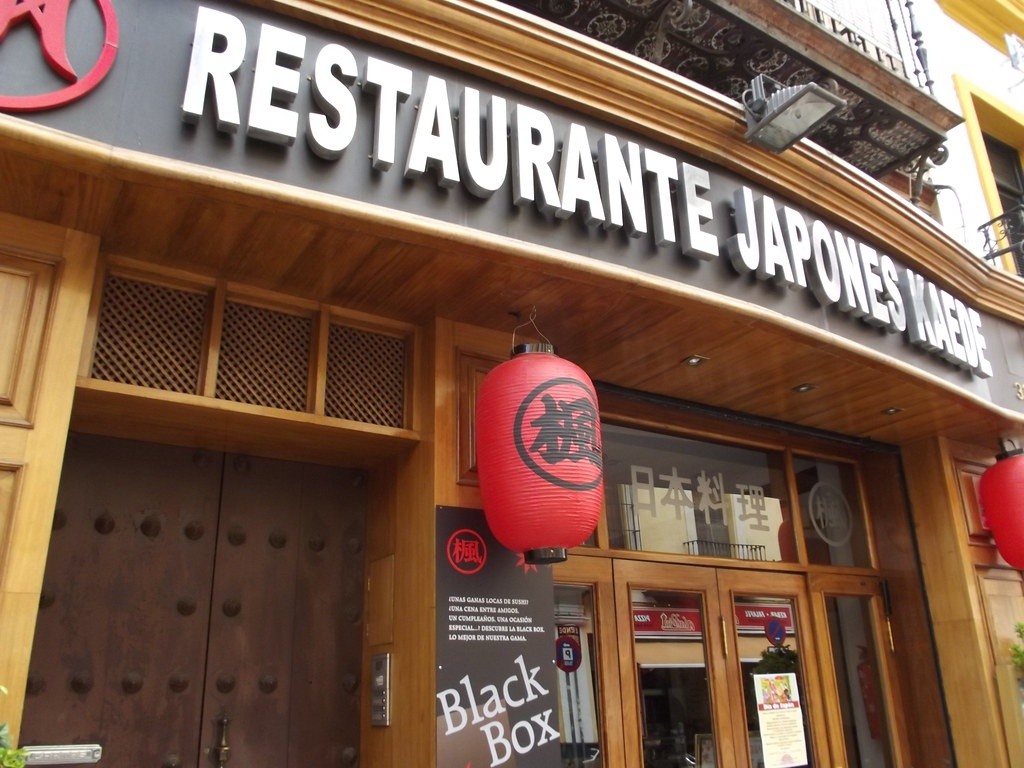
xmin=742 ymin=74 xmax=847 ymax=154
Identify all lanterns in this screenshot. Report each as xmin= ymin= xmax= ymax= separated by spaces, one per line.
xmin=977 ymin=448 xmax=1024 ymax=572
xmin=474 ymin=342 xmax=604 ymax=565
xmin=778 ymin=518 xmax=800 ymax=563
